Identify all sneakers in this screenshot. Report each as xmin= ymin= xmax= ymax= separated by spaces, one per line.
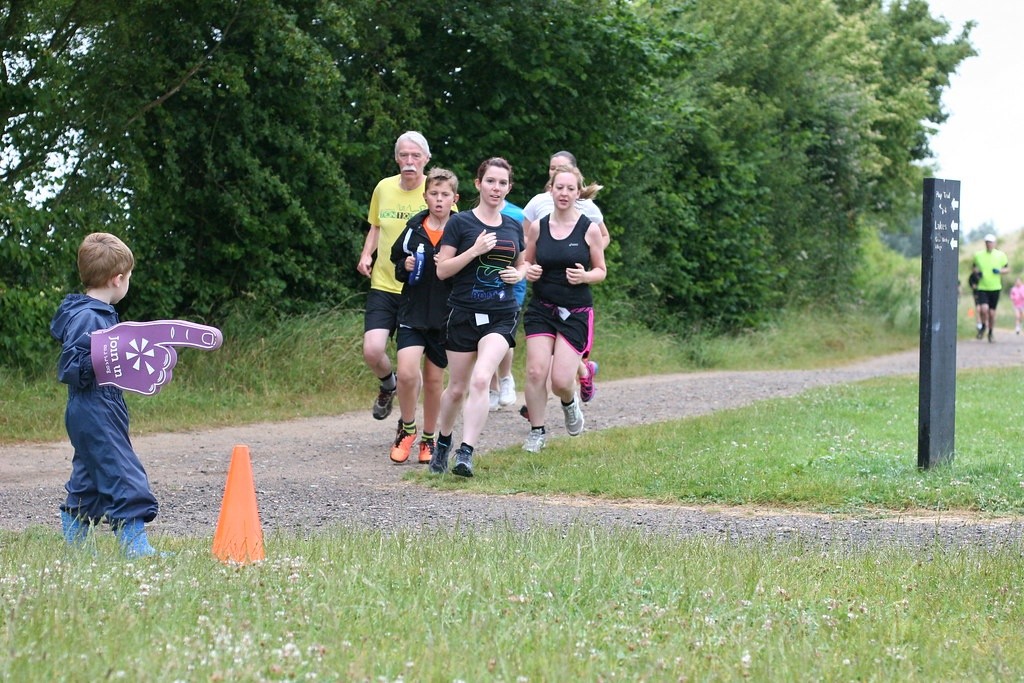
xmin=522 ymin=428 xmax=546 ymax=454
xmin=429 ymin=434 xmax=454 ymax=473
xmin=396 ymin=418 xmax=403 ymax=439
xmin=561 ymin=391 xmax=584 ymax=436
xmin=488 ymin=390 xmax=502 ymax=412
xmin=390 ymin=426 xmax=418 ymax=463
xmin=520 ymin=404 xmax=530 ymax=421
xmin=419 ymin=441 xmax=436 ymax=464
xmin=372 ymin=372 xmax=397 ymax=420
xmin=452 ymin=445 xmax=473 ymax=477
xmin=579 ymin=360 xmax=599 ymax=402
xmin=498 ymin=373 xmax=516 ymax=406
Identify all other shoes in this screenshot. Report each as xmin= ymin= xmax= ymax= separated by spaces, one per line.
xmin=988 ymin=333 xmax=994 ymax=343
xmin=977 ymin=328 xmax=984 ymax=339
xmin=977 ymin=322 xmax=983 ymax=330
xmin=1016 ymin=327 xmax=1020 ymax=335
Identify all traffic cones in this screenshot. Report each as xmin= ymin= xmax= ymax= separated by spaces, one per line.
xmin=210 ymin=443 xmax=265 ymax=563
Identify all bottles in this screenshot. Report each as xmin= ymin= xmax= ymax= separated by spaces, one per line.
xmin=408 ymin=243 xmax=424 ymax=286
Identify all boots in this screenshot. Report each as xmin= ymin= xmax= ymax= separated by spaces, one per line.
xmin=61 ymin=510 xmax=89 ymax=549
xmin=114 ymin=517 xmax=177 ymax=561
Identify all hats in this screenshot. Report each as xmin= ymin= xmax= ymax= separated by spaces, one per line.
xmin=984 ymin=234 xmax=996 ymax=241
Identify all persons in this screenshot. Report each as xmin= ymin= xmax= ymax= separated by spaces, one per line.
xmin=389 ymin=169 xmax=460 ymax=463
xmin=967 ymin=234 xmax=1009 ymax=342
xmin=357 ymin=130 xmax=459 ymax=419
xmin=522 ymin=167 xmax=607 ymax=452
xmin=49 ymin=233 xmax=222 ymax=556
xmin=428 ymin=158 xmax=529 ymax=475
xmin=520 ymin=151 xmax=611 ymax=421
xmin=488 ymin=199 xmax=525 ymax=411
xmin=1010 ymin=278 xmax=1024 ymax=334
